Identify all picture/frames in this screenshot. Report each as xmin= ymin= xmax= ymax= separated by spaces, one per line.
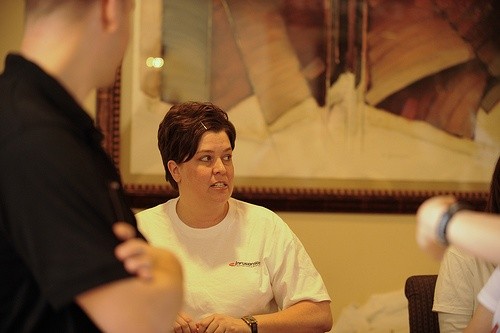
xmin=95 ymin=0 xmax=500 ymax=215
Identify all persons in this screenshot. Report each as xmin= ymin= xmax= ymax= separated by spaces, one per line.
xmin=134 ymin=101 xmax=333 ymax=333
xmin=416 ymin=156 xmax=500 ymax=333
xmin=0 ymin=0 xmax=184 ymax=333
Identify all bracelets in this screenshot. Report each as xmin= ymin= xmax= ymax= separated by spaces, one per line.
xmin=436 ymin=205 xmax=466 ymax=248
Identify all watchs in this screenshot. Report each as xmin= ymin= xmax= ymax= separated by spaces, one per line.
xmin=240 ymin=316 xmax=258 ymax=333
